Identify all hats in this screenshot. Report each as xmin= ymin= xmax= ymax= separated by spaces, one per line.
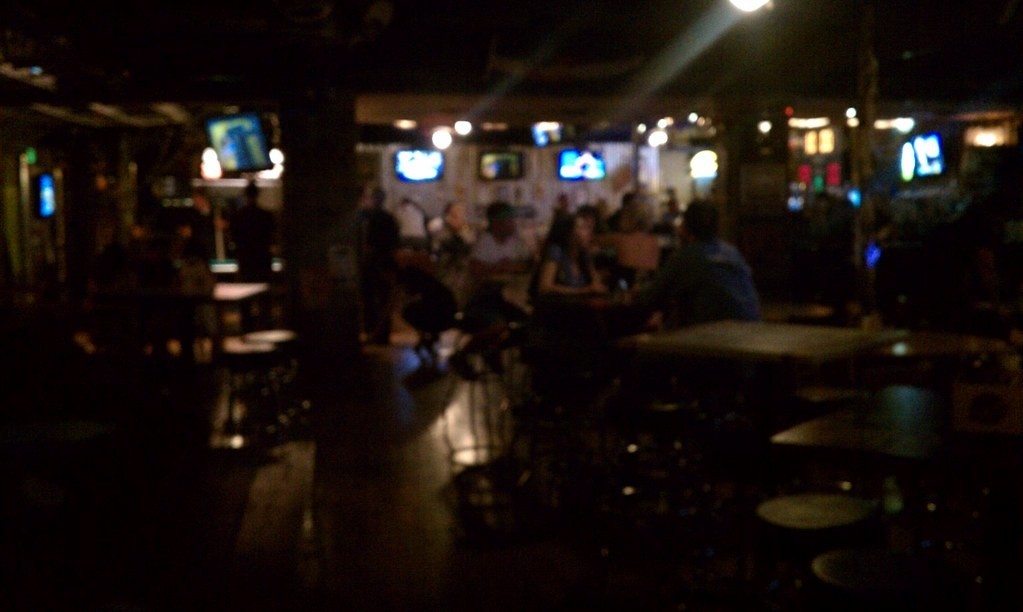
xmin=484 ymin=202 xmax=510 ymax=231
xmin=865 ymin=385 xmax=935 ymax=457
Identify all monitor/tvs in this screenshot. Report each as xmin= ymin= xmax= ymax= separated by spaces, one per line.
xmin=896 ymin=131 xmax=947 ymax=182
xmin=557 ymin=149 xmax=606 ymax=180
xmin=36 ymin=173 xmax=57 ymax=220
xmin=475 ymin=150 xmax=525 ymax=180
xmin=394 ymin=149 xmax=445 ymax=182
xmin=203 ymin=110 xmax=274 ymax=178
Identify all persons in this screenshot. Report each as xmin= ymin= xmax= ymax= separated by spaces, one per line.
xmin=355 ymin=189 xmax=856 ymax=380
xmin=95 ymin=183 xmax=278 ymax=332
xmin=871 ymin=125 xmax=1023 ymax=332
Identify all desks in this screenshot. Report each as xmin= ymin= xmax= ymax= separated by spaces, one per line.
xmin=630 ymin=318 xmax=910 ymax=426
xmin=548 ymin=291 xmax=646 ymax=377
xmin=210 ymin=282 xmax=270 ymax=333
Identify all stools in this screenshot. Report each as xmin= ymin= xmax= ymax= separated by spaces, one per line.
xmin=219 ymin=329 xmax=297 ymax=435
xmin=756 ymin=496 xmax=870 ymax=612
xmin=458 ymin=312 xmax=514 ymax=399
